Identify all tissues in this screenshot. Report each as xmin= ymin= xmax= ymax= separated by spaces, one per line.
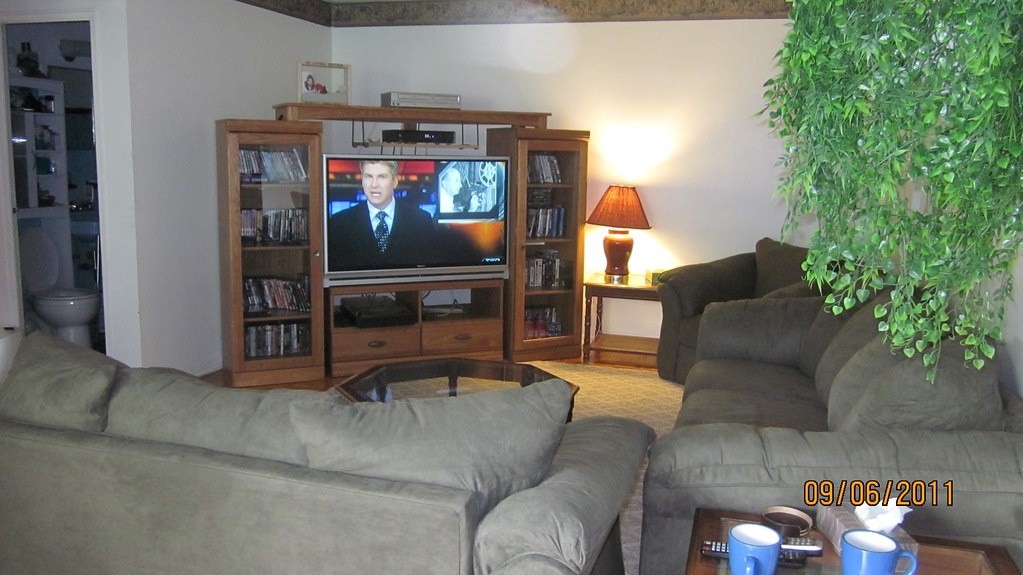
xmin=815 ymin=497 xmax=918 ymax=572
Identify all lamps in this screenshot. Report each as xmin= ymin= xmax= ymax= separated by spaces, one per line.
xmin=585 ymin=185 xmax=650 ymax=278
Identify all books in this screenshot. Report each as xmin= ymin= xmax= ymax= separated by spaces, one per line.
xmin=241 ymin=276 xmax=312 ymax=320
xmin=242 ymin=323 xmax=310 ymax=361
xmin=525 ymin=245 xmax=562 ymax=287
xmin=527 ymin=153 xmax=562 ymax=184
xmin=241 ymin=204 xmax=309 ymax=246
xmin=523 ymin=303 xmax=563 ymax=340
xmin=237 ymin=144 xmax=308 ymax=183
xmin=526 ymin=186 xmax=566 ymax=239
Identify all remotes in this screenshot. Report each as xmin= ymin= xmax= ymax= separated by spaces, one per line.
xmin=782 ymin=538 xmax=824 ymax=557
xmin=701 ymin=539 xmax=808 ymax=568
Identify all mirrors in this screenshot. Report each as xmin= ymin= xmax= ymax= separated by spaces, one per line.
xmin=66 ymin=107 xmax=94 ymax=149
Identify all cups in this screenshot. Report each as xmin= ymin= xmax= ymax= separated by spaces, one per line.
xmin=728 ymin=523 xmax=781 ymax=574
xmin=840 ymin=529 xmax=916 ymax=575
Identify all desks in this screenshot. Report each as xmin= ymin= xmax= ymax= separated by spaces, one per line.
xmin=684 ymin=508 xmax=1021 ymax=574
xmin=582 ymin=271 xmax=663 ymax=365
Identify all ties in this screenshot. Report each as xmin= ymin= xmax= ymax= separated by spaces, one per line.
xmin=372 ymin=211 xmax=391 ymax=253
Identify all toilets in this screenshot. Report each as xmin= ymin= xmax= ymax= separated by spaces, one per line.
xmin=18 ymin=227 xmax=101 ymax=348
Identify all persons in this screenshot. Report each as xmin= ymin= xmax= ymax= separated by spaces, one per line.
xmin=441 ymin=164 xmax=466 ymax=214
xmin=328 ymin=158 xmax=453 ymax=267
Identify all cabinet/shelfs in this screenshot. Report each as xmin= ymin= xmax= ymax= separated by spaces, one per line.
xmin=6 ymin=74 xmax=75 ymax=291
xmin=215 ymin=101 xmax=591 ymax=388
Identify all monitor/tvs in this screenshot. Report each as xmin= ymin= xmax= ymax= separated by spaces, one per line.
xmin=323 ymin=153 xmax=509 ymax=288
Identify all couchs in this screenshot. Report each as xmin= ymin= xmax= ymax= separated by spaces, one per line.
xmin=638 ymin=239 xmax=1023 ymax=575
xmin=0 ymin=327 xmax=659 ymax=575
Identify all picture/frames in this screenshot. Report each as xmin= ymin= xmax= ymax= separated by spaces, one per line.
xmin=298 ymin=63 xmax=350 ymax=105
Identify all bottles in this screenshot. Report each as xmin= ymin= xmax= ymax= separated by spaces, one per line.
xmin=17 ymin=42 xmax=39 ymax=72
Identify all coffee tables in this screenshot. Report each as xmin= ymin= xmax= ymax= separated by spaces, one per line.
xmin=336 ymin=357 xmax=580 ymax=422
